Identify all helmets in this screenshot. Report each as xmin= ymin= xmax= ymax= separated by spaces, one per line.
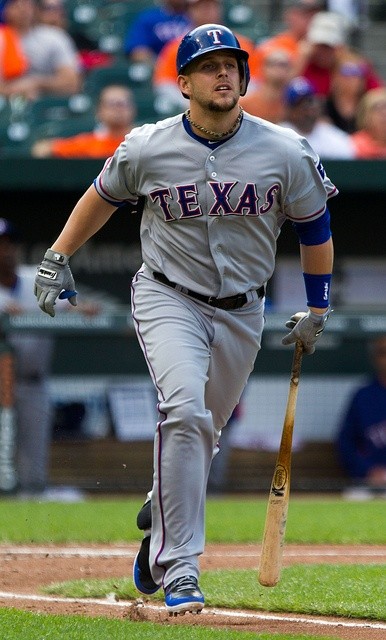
xmin=176 ymin=23 xmax=250 ymax=98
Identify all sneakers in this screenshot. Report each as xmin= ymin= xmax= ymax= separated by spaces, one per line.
xmin=133 ymin=535 xmax=161 ymax=596
xmin=165 ymin=576 xmax=206 ymax=616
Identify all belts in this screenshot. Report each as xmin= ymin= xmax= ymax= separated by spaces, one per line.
xmin=153 ymin=272 xmax=264 ymax=310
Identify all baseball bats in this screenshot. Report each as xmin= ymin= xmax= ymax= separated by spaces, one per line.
xmin=258 ymin=312 xmax=308 ymax=587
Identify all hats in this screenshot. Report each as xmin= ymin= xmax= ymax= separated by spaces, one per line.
xmin=308 ymin=10 xmax=346 ymax=49
xmin=287 ymin=77 xmax=317 ymax=109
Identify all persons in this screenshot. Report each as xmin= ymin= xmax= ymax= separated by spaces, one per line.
xmin=33 ymin=22 xmax=341 ymax=616
xmin=335 ymin=338 xmax=386 ymax=494
xmin=125 ymin=0 xmax=190 ymax=67
xmin=154 ymin=0 xmax=255 ymax=109
xmin=34 ymin=84 xmax=138 ymax=157
xmin=0 ymin=1 xmax=81 ymax=97
xmin=38 ymin=1 xmax=92 ymax=71
xmin=250 ymin=2 xmax=386 ymax=160
xmin=0 ymin=219 xmax=49 ymax=496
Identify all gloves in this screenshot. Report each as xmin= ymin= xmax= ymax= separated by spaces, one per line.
xmin=281 ymin=304 xmax=334 ymax=355
xmin=32 ymin=248 xmax=78 ymax=318
